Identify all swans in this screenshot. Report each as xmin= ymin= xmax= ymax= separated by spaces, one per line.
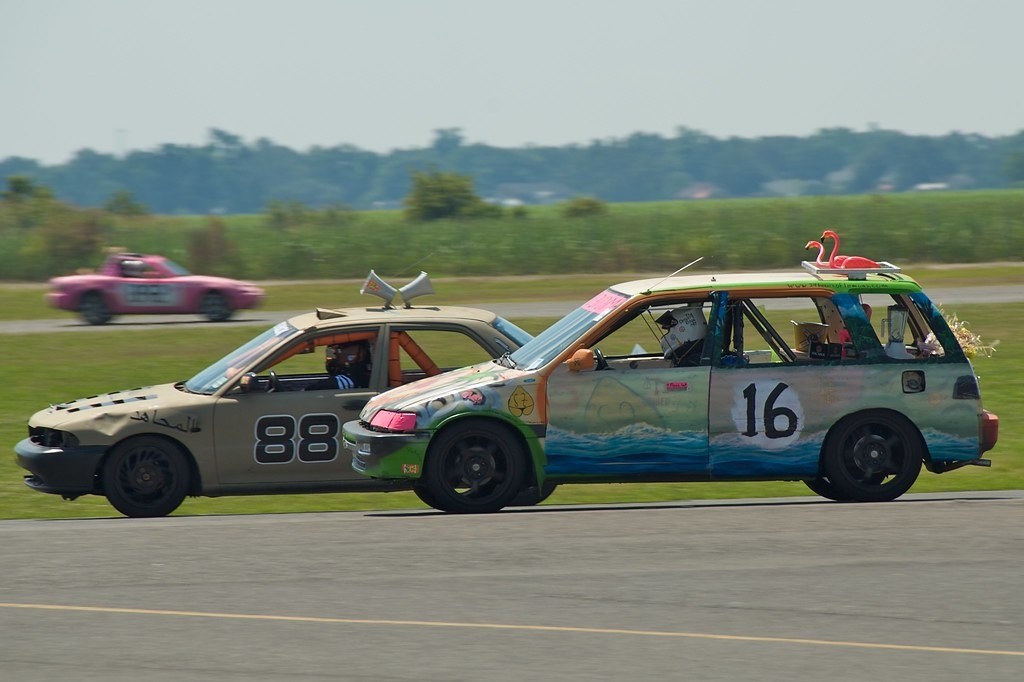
xmin=821 ymin=230 xmax=881 ymax=268
xmin=805 ymin=240 xmax=848 ymax=268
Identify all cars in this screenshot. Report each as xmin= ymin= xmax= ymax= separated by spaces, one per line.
xmin=343 ymin=270 xmax=998 ymax=515
xmin=13 ymin=306 xmax=535 ymax=520
xmin=46 ymin=256 xmax=267 ymax=326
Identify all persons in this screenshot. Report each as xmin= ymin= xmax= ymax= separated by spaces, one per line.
xmin=603 ymin=305 xmax=708 ymax=371
xmin=301 ymin=339 xmax=372 ymax=392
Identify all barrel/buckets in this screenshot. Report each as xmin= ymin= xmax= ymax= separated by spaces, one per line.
xmin=793 ymin=322 xmax=829 ymax=352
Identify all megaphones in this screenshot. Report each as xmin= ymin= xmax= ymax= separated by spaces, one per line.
xmin=397 ymin=270 xmax=435 ymax=302
xmin=360 ymin=269 xmax=398 ymax=303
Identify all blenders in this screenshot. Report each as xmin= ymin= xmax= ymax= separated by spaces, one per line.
xmin=880 ymin=305 xmax=914 ymax=359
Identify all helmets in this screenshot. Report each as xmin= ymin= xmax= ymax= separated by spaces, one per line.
xmin=325 ymin=339 xmax=375 ymax=376
xmin=654 ymin=307 xmax=708 ymax=361
